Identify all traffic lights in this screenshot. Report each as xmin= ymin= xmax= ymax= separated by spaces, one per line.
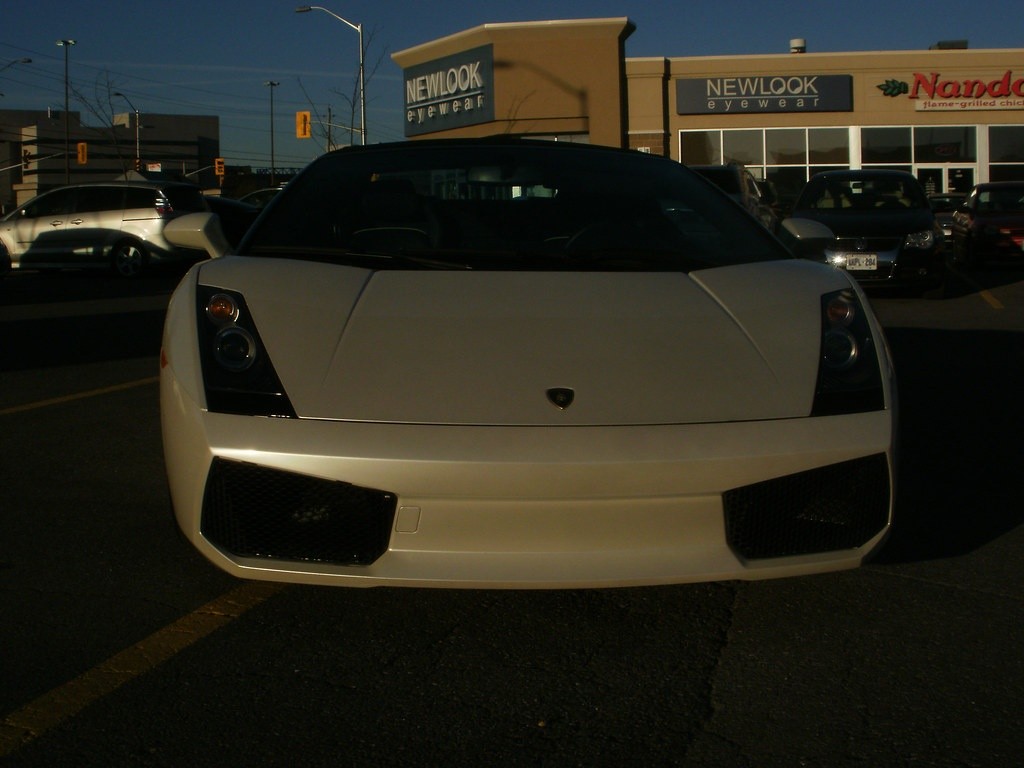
xmin=135 ymin=158 xmax=140 ymax=172
xmin=215 ymin=158 xmax=225 ymax=175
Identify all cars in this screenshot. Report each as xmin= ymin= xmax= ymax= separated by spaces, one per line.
xmin=160 ymin=136 xmax=903 ymax=590
xmin=952 ymin=181 xmax=1024 ymax=269
xmin=237 ymin=187 xmax=283 ymax=207
xmin=204 ymin=195 xmax=259 ymax=243
xmin=793 ymin=169 xmax=945 ymax=290
xmin=925 ymin=192 xmax=981 ymax=242
xmin=665 ymin=163 xmax=776 ymax=244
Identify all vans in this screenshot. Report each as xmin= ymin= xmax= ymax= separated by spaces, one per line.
xmin=0 ymin=182 xmax=213 ymax=278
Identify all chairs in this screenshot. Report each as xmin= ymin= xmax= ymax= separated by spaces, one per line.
xmin=875 ymin=181 xmax=910 ymax=208
xmin=536 ymin=177 xmax=633 ymax=238
xmin=982 ymin=190 xmax=1006 ymax=210
xmin=333 ymin=179 xmax=445 ymax=246
xmin=819 ymin=185 xmax=852 ymax=208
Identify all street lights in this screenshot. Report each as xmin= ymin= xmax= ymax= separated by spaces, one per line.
xmin=295 ymin=5 xmax=366 ymax=146
xmin=57 ymin=40 xmax=76 ymax=184
xmin=263 ymin=81 xmax=280 ymax=188
xmin=112 ymin=92 xmax=140 ymax=174
